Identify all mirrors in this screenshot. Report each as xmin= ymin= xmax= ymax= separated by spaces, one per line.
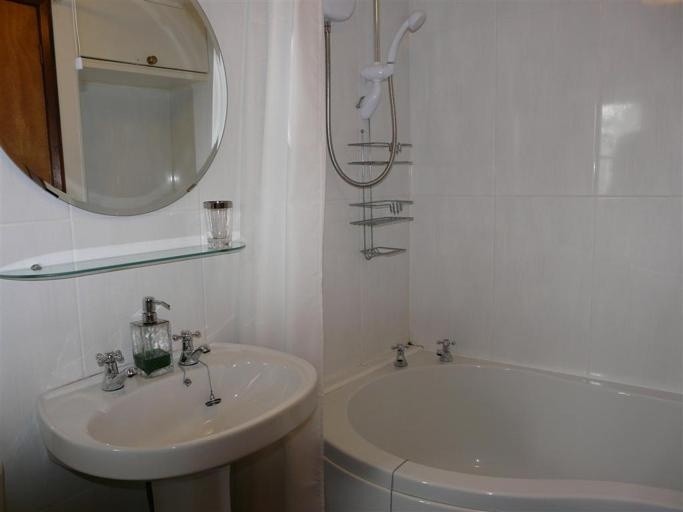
xmin=0 ymin=1 xmax=230 ymax=218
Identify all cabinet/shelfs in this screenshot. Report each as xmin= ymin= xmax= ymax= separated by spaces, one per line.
xmin=74 ymin=2 xmax=209 ymax=79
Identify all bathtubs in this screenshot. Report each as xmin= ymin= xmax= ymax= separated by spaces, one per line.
xmin=323 ymin=352 xmax=683 ymax=512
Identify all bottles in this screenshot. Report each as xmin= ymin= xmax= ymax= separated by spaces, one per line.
xmin=204 ymin=200 xmax=232 ymax=252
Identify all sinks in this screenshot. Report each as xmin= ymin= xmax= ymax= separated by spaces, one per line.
xmin=39 ymin=342 xmax=318 ymax=481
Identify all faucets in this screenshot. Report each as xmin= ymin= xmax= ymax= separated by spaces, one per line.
xmin=392 ymin=344 xmax=407 ymax=367
xmin=435 ymin=338 xmax=456 ymax=362
xmin=173 ymin=328 xmax=212 ymax=365
xmin=97 ymin=349 xmax=136 ymax=392
